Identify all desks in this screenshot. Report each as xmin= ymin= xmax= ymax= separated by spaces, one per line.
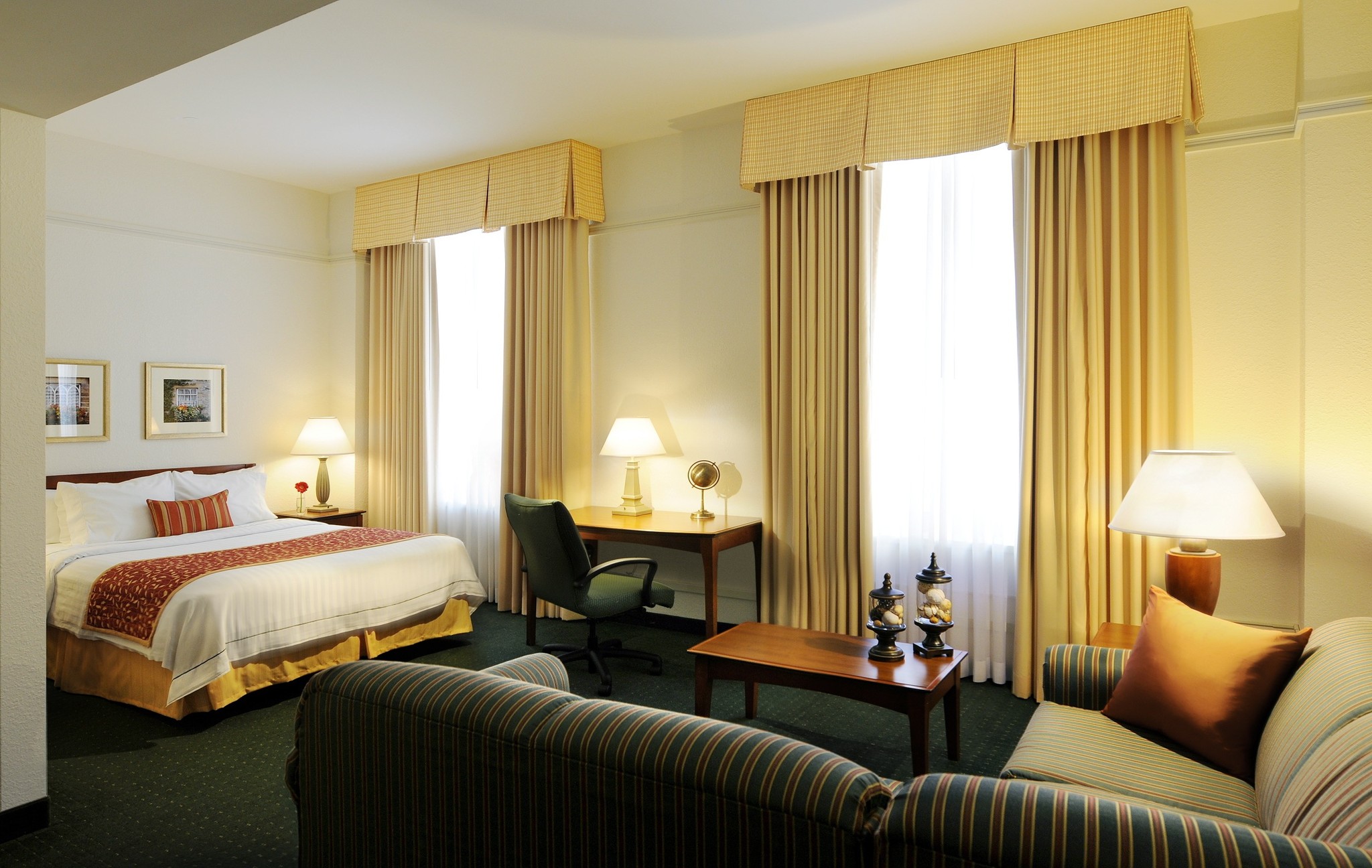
xmin=521 ymin=505 xmax=763 ymax=667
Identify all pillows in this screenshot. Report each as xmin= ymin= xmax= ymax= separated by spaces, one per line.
xmin=1100 ymin=586 xmax=1312 ymax=779
xmin=55 ymin=463 xmax=279 ymax=546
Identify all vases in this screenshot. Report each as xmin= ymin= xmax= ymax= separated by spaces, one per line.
xmin=297 ymin=492 xmax=305 ymax=514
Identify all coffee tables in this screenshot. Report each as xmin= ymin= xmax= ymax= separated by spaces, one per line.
xmin=688 ymin=620 xmax=969 ymax=777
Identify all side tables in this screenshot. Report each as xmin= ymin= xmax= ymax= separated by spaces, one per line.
xmin=1093 ymin=623 xmax=1142 ymax=650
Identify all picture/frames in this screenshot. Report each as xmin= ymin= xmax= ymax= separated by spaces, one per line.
xmin=145 ymin=362 xmax=228 ymax=440
xmin=46 ymin=359 xmax=110 ymax=443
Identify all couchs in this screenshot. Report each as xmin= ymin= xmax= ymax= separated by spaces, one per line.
xmin=289 ymin=616 xmax=1372 ymax=868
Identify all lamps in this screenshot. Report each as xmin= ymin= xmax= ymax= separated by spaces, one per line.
xmin=290 ymin=417 xmax=355 ymax=513
xmin=600 ymin=417 xmax=667 ymax=516
xmin=1109 ymin=448 xmax=1286 ymax=614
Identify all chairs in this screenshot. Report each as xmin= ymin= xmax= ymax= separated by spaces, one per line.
xmin=504 ymin=493 xmax=675 ymax=695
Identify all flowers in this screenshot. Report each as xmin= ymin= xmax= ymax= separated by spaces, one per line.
xmin=295 ymin=482 xmax=308 ymax=513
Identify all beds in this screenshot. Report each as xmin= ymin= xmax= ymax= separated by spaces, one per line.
xmin=45 ymin=463 xmax=490 ymax=720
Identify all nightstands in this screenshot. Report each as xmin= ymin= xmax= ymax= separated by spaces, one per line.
xmin=275 ymin=508 xmax=367 ymax=527
